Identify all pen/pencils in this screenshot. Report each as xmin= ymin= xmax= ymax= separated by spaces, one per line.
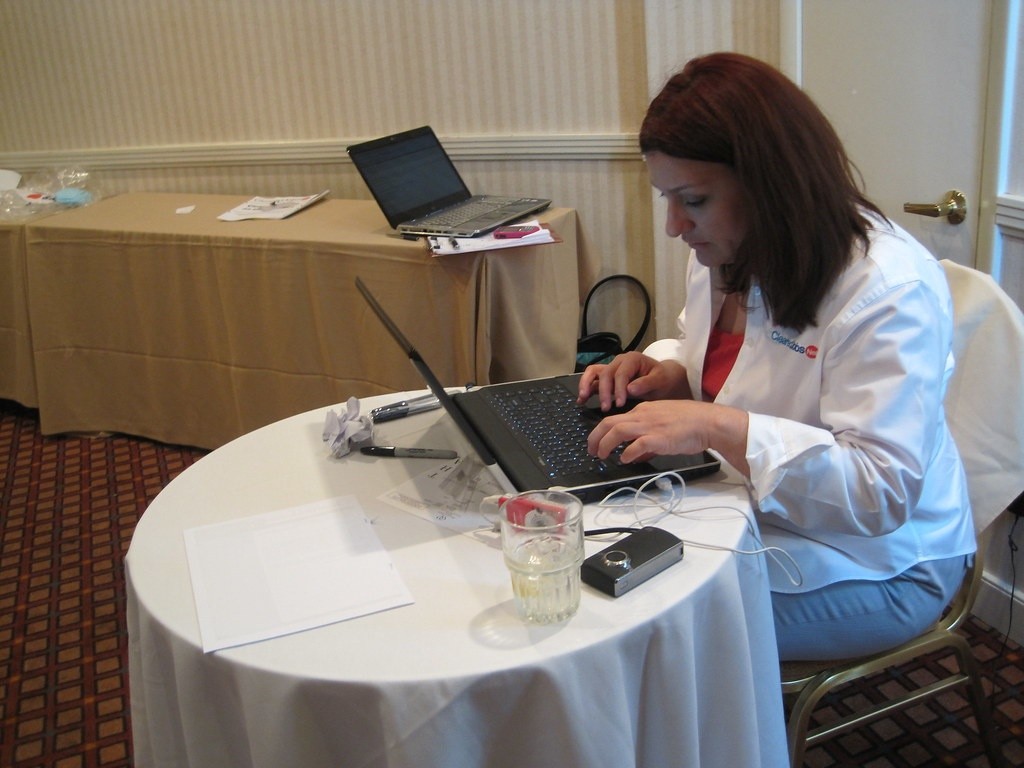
xmin=449 ymin=236 xmax=460 ymax=250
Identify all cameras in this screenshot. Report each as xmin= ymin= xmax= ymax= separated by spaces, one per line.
xmin=580 ymin=526 xmax=685 ymax=598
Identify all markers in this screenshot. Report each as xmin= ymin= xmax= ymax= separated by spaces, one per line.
xmin=360 ymin=446 xmax=458 ymax=460
xmin=371 ymin=392 xmax=442 ymax=424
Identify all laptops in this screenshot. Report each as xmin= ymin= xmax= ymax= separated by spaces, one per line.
xmin=346 ymin=124 xmax=552 ymax=238
xmin=352 ymin=275 xmax=721 ymax=503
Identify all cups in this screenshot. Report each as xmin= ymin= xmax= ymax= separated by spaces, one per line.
xmin=498 ymin=489 xmax=586 ymax=627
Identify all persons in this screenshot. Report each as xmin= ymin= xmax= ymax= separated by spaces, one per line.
xmin=577 ymin=53 xmax=977 ymax=662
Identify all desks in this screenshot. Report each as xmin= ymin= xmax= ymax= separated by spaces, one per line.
xmin=25 ymin=188 xmax=588 ymax=452
xmin=122 ymin=386 xmax=790 ymax=768
xmin=0 ymin=193 xmax=113 ymax=410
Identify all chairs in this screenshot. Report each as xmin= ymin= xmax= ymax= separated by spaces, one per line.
xmin=780 ymin=259 xmax=1024 ymax=768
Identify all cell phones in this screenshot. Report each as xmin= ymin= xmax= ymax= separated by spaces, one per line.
xmin=493 ymin=225 xmax=540 ymax=238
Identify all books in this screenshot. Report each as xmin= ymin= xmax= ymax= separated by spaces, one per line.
xmin=217 ymin=190 xmax=330 ymax=221
